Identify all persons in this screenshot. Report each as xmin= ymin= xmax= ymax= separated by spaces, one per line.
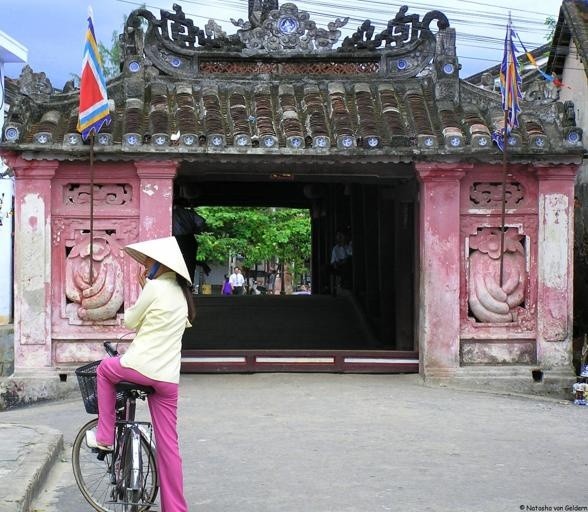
xmin=85 ymin=255 xmax=196 ymax=512
xmin=330 ymin=226 xmax=352 ymax=290
xmin=172 ymin=197 xmax=212 ymax=290
xmin=221 ymin=266 xmax=246 ymax=297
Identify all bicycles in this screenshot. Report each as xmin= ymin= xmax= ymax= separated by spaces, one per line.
xmin=72 ymin=332 xmax=160 ymax=512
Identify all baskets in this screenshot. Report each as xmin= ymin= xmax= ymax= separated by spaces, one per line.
xmin=74 ymin=359 xmax=125 ymax=414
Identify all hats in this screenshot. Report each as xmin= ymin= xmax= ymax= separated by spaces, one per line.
xmin=123 ymin=236 xmax=192 ymax=288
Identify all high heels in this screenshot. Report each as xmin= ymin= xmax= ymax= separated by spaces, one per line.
xmin=85 ymin=430 xmax=110 ymax=461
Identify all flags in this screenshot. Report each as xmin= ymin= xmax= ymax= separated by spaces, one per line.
xmin=492 ymin=27 xmax=522 ymax=152
xmin=76 ymin=16 xmax=114 ymax=142
